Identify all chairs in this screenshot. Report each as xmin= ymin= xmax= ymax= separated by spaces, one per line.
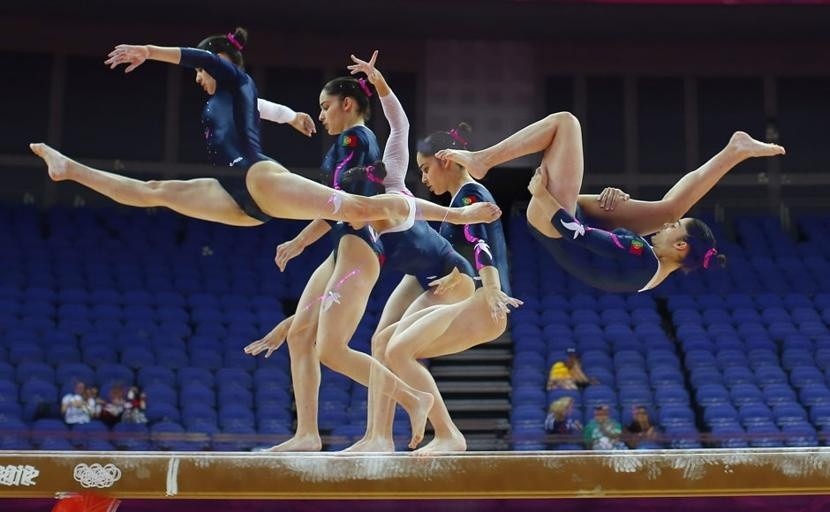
xmin=0 ymin=200 xmax=420 ymax=454
xmin=507 ymin=199 xmax=828 ymax=447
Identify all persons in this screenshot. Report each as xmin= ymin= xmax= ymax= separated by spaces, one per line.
xmin=433 ymin=110 xmax=788 ymax=296
xmin=261 ymin=75 xmax=437 ymax=453
xmin=240 ymin=49 xmax=475 ymax=452
xmin=342 ymin=121 xmax=525 ymax=451
xmin=545 ymin=347 xmax=663 ymax=450
xmin=59 ymin=380 xmax=150 ymax=425
xmin=28 ymin=29 xmax=505 ymax=230
